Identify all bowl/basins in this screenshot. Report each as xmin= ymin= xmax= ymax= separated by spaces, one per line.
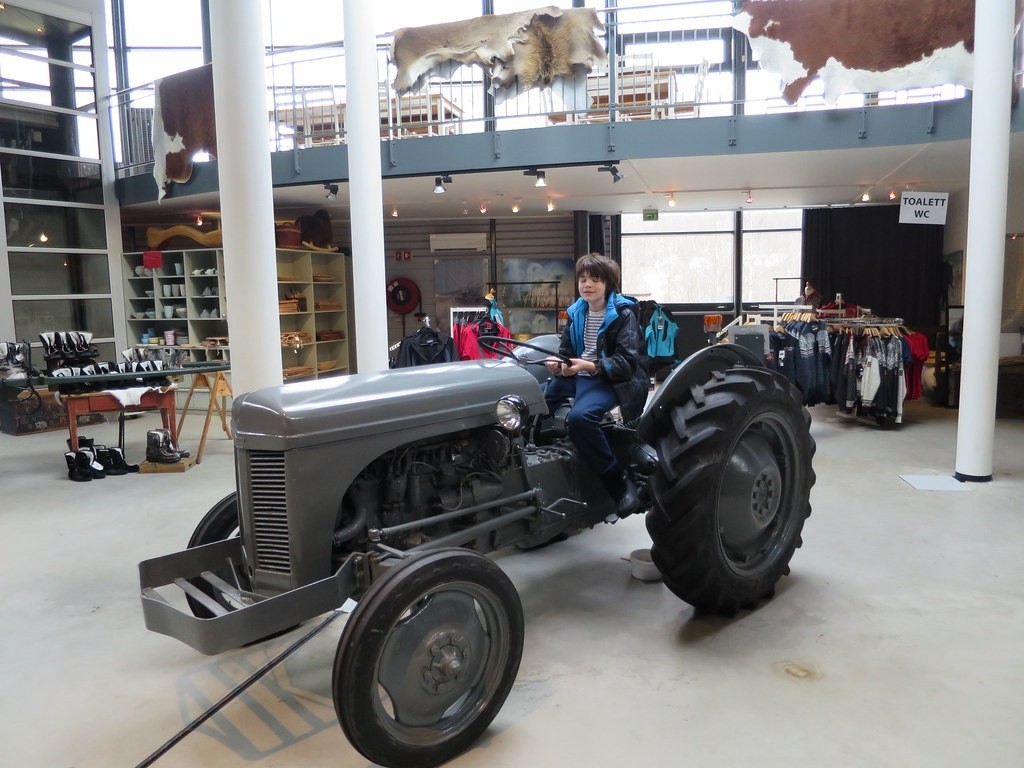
xmin=630 ymin=548 xmax=662 ymax=580
xmin=176 ymin=308 xmax=187 ymax=318
xmin=135 ymin=266 xmax=152 ymax=277
xmin=132 ymin=312 xmax=144 ymax=319
xmin=145 ymin=312 xmax=155 ymax=319
xmin=145 ymin=291 xmax=154 ymax=297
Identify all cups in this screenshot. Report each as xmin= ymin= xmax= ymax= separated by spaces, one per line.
xmin=180 ymin=284 xmax=185 ymax=296
xmin=172 ymin=284 xmax=179 ymax=296
xmin=165 ymin=331 xmax=175 ymax=345
xmin=175 ymin=264 xmax=183 ymax=275
xmin=164 ymin=306 xmax=173 ymax=318
xmin=163 ymin=285 xmax=171 ymax=297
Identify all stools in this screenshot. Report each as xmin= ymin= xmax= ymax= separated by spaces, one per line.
xmin=176 ymin=360 xmax=233 ymax=464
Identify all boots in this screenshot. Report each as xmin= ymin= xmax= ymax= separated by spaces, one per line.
xmin=0 ymin=343 xmax=10 ymax=370
xmin=91 ymin=444 xmax=106 ymax=458
xmin=147 ymin=430 xmax=181 ymax=463
xmin=9 ymin=342 xmax=24 ymax=366
xmin=65 ymin=451 xmax=93 ymax=481
xmin=39 ymin=328 xmax=170 ymax=392
xmin=78 ymin=447 xmax=90 ymax=451
xmin=97 ymin=449 xmax=125 ymax=475
xmin=601 ymin=465 xmax=639 ymax=519
xmin=156 ymin=429 xmax=190 ymax=458
xmin=108 ymin=447 xmax=139 ymax=472
xmin=77 ymin=446 xmax=105 ymax=478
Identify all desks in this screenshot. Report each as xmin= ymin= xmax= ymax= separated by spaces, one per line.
xmin=58 ymin=383 xmax=179 ymax=454
xmin=587 ymin=71 xmax=678 ymax=120
xmin=268 ymin=93 xmax=465 ymax=137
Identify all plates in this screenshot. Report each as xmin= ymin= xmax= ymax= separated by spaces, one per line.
xmin=177 ymin=337 xmax=188 ymax=345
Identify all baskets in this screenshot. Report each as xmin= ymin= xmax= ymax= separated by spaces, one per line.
xmin=276 ymin=222 xmax=302 ymax=248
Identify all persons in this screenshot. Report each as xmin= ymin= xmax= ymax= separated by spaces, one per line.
xmin=793 ymin=285 xmax=825 ymax=312
xmin=544 ymin=253 xmax=649 ymax=518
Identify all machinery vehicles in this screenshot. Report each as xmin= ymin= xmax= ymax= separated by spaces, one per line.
xmin=137 ymin=332 xmax=817 ymax=768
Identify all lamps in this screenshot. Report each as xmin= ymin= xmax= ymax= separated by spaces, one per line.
xmin=432 ymin=177 xmax=453 ymax=194
xmin=524 ymin=171 xmax=546 ymax=187
xmin=598 ymin=167 xmax=625 ymax=183
xmin=325 ymin=185 xmax=338 ymax=201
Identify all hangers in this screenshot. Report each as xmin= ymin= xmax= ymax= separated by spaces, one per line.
xmin=861 ymin=318 xmax=915 ymax=338
xmin=456 ymin=311 xmax=498 ymax=335
xmin=826 ymin=319 xmax=856 ymax=335
xmin=773 ymin=325 xmax=785 ymax=336
xmin=639 ymin=294 xmax=659 ymax=308
xmin=781 ymin=306 xmax=819 ymax=324
xmin=418 ymin=326 xmax=438 ymax=347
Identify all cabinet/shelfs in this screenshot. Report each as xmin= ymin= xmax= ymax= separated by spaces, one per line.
xmin=120 ymin=248 xmax=233 ymax=418
xmin=275 ymin=248 xmax=349 ymax=384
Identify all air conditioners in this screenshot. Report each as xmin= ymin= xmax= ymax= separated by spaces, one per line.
xmin=429 ymin=233 xmax=487 ymax=253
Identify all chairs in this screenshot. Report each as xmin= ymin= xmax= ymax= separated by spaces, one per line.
xmin=275 ymin=81 xmax=438 ymax=151
xmin=664 ymin=58 xmax=711 ymax=120
xmin=541 ymin=52 xmax=659 ymax=126
